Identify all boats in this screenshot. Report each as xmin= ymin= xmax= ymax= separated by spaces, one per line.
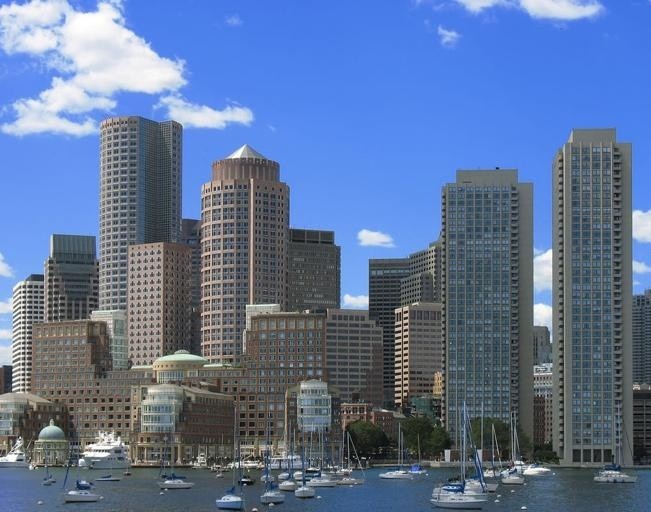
xmin=75 ymin=480 xmax=95 ymax=490
xmin=156 ymin=478 xmax=196 ymax=490
xmin=95 ymin=473 xmax=122 ymax=482
xmin=0 ymin=436 xmax=30 ymax=468
xmin=64 ymin=490 xmax=103 ymax=502
xmin=124 ymin=467 xmax=131 ymax=477
xmin=81 ymin=430 xmax=132 ymax=470
xmin=523 ymin=463 xmax=551 ymax=475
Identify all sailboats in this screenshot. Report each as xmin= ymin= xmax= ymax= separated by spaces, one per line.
xmin=378 ymin=421 xmax=427 ymax=479
xmin=592 ymin=399 xmax=641 ymax=484
xmin=160 ymin=434 xmax=186 ymax=480
xmin=430 ymin=400 xmax=530 ymax=508
xmin=41 ymin=441 xmax=56 ymax=487
xmin=193 ymin=414 xmax=366 ymax=509
